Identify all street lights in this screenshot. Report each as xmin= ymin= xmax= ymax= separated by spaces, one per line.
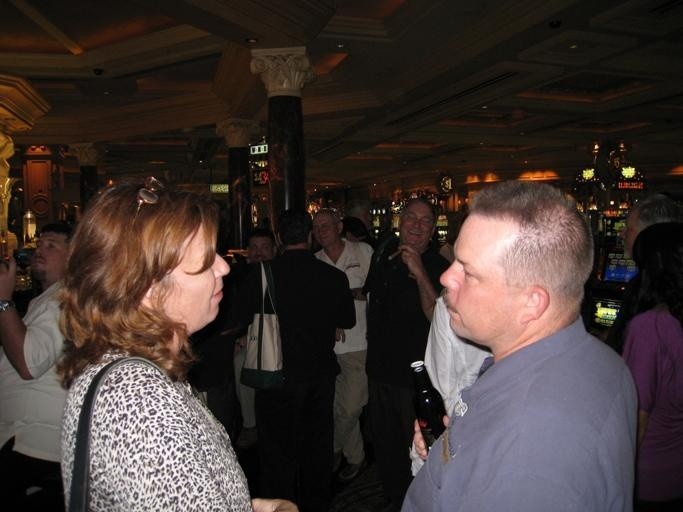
xmin=239 ymin=313 xmax=283 ymax=389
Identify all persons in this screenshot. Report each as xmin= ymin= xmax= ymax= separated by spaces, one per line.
xmin=400 ymin=178 xmax=638 ymax=510
xmin=2 ymin=218 xmax=80 ymax=512
xmin=604 ymin=193 xmax=680 ymax=355
xmin=57 ymin=175 xmax=299 ymax=512
xmin=186 ymin=196 xmax=486 ymax=510
xmin=622 ymin=221 xmax=682 ymax=510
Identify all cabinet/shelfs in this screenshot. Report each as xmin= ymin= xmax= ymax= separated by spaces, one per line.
xmin=410 ymin=360 xmax=448 ymax=453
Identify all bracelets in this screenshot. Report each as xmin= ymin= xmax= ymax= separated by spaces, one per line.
xmin=2 ymin=300 xmax=16 ymax=313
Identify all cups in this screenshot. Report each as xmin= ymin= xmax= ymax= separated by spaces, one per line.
xmin=131 ymin=175 xmax=166 ymax=223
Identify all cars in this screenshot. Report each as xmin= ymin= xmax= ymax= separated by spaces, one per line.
xmin=272 ymin=500 xmax=298 ymax=512
